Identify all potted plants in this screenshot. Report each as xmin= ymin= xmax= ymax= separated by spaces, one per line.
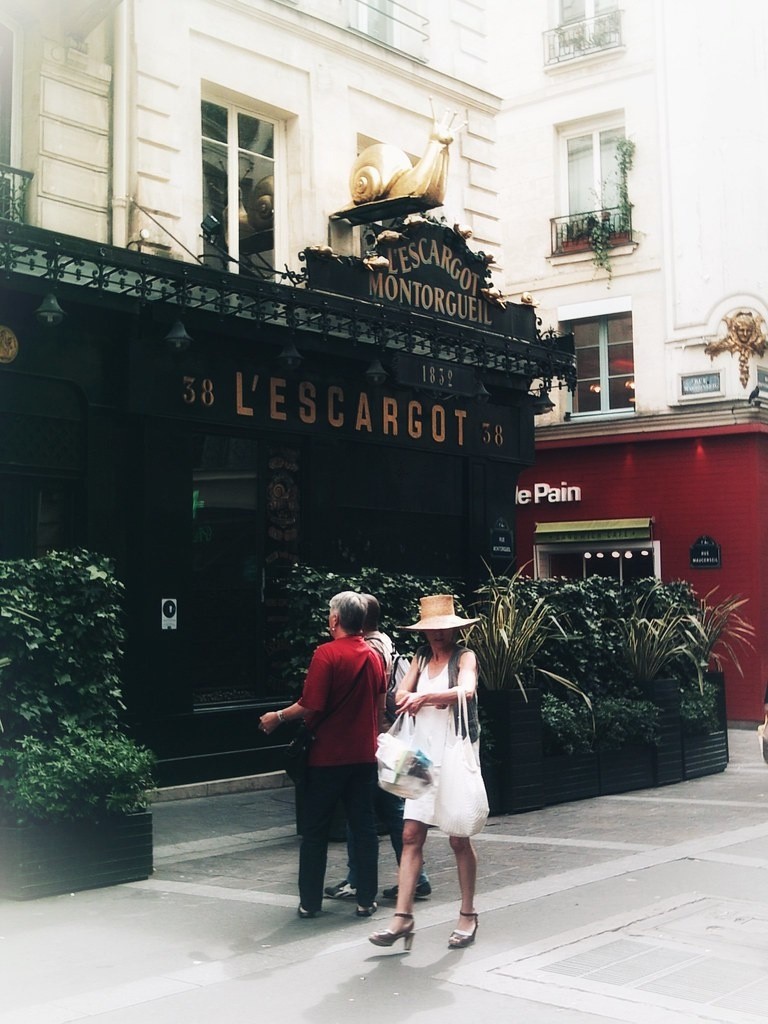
xmin=561 ymin=212 xmax=631 ymax=290
xmin=294 ymin=555 xmax=758 ymax=844
xmin=0 ymin=722 xmax=163 ymax=902
xmin=587 ymin=178 xmax=611 ymax=222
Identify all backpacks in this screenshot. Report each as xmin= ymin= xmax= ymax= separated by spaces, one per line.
xmin=365 ymin=638 xmax=411 ymax=729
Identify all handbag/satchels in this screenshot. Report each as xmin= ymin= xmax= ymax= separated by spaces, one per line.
xmin=375 ymin=710 xmax=434 ymax=800
xmin=284 ymin=728 xmax=316 ymax=784
xmin=435 ymin=686 xmax=489 ymax=837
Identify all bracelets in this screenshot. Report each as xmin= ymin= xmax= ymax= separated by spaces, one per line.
xmin=276 ymin=710 xmax=286 ymax=722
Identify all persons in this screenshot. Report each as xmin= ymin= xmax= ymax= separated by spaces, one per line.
xmin=368 ymin=594 xmax=489 ymax=950
xmin=257 ymin=593 xmax=388 ymax=919
xmin=702 ymin=311 xmax=768 ymax=389
xmin=324 ymin=591 xmax=432 ymax=901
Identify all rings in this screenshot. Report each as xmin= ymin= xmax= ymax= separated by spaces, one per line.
xmin=263 ymin=729 xmax=266 ymax=732
xmin=412 ymin=712 xmax=415 ymax=717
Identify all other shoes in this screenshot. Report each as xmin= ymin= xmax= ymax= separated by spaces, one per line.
xmin=358 ymin=900 xmax=378 ymax=916
xmin=298 ymin=903 xmax=316 ymax=918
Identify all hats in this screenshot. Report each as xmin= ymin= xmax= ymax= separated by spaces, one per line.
xmin=405 ymin=595 xmax=481 ymax=631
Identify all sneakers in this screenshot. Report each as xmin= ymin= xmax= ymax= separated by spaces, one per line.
xmin=325 ymin=881 xmax=358 ymax=899
xmin=383 ymin=881 xmax=432 ymax=899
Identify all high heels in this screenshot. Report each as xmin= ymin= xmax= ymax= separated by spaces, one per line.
xmin=449 ymin=908 xmax=479 ymax=947
xmin=369 ymin=914 xmax=415 ymax=950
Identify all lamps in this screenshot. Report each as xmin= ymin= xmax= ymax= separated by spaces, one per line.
xmin=470 ymin=379 xmax=492 ymax=407
xmin=199 ymin=214 xmax=257 ymax=278
xmin=533 ymin=392 xmax=555 ymax=414
xmin=275 ymin=339 xmax=305 ymax=371
xmin=164 ymin=320 xmax=195 ymax=354
xmin=31 ymin=291 xmax=68 ymax=329
xmin=364 ymin=355 xmax=391 ymax=386
